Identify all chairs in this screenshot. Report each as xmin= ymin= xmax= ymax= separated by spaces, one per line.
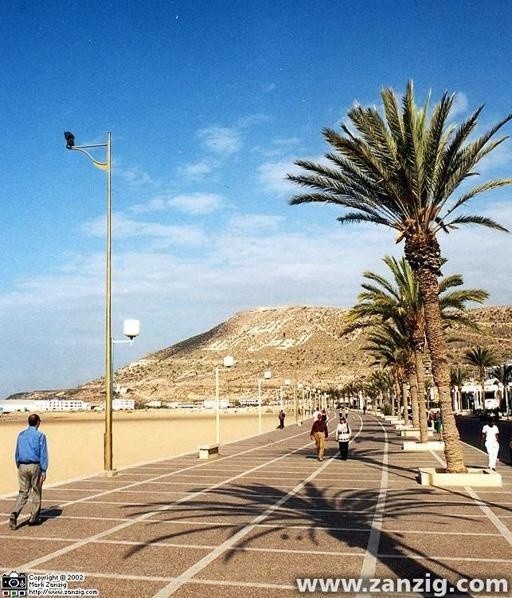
xmin=9 ymin=512 xmax=18 ymax=530
xmin=28 ymin=519 xmax=42 ymax=527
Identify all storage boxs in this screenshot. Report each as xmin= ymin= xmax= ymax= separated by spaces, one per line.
xmin=198 ymin=443 xmax=219 ymax=458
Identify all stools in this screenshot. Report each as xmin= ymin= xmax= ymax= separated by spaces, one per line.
xmin=19 ymin=461 xmax=40 ymax=465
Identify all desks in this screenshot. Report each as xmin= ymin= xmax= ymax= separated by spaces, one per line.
xmin=62 ymin=125 xmax=144 ymax=472
xmin=258 ymin=371 xmax=273 ymax=433
xmin=279 ymin=379 xmax=329 ymax=427
xmin=215 ymin=354 xmax=236 ymax=444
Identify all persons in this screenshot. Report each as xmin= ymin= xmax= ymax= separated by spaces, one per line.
xmin=335 ymin=416 xmax=352 ymax=462
xmin=480 ymin=415 xmax=501 ymax=471
xmin=363 ymin=405 xmax=366 ymax=413
xmin=313 ymin=405 xmax=350 ymax=430
xmin=9 ymin=412 xmax=50 ymax=531
xmin=309 ymin=413 xmax=329 ymax=461
xmin=278 ymin=409 xmax=285 ymax=427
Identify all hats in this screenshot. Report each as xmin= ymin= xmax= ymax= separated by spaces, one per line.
xmin=401 ymin=401 xmax=441 ymax=425
xmin=479 ymin=409 xmax=499 ymax=422
